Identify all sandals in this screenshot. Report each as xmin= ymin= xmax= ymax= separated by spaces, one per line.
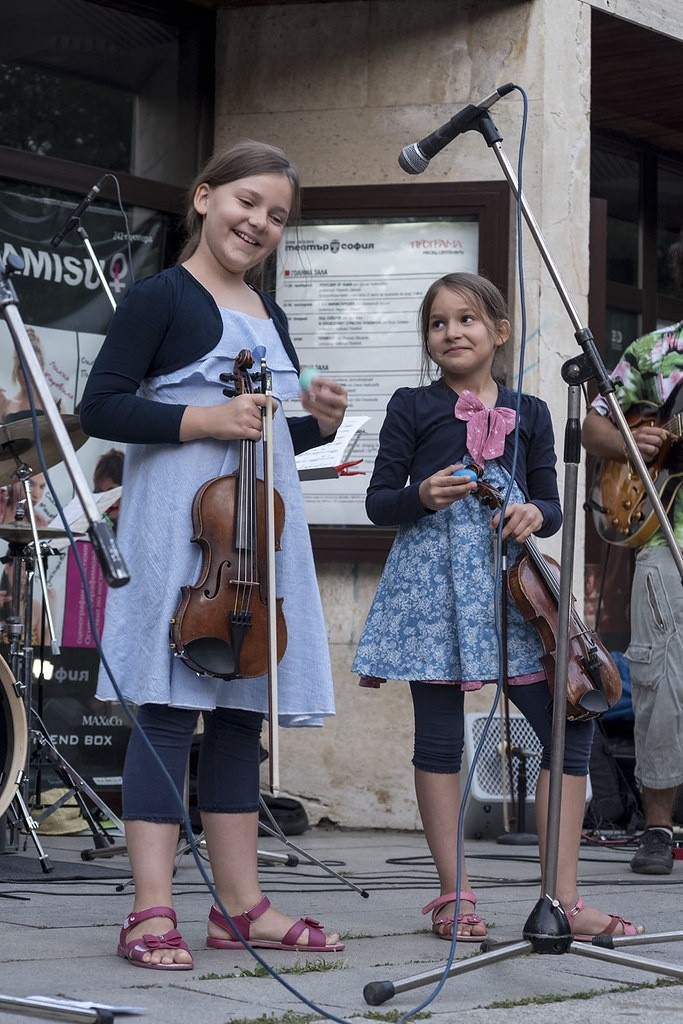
xmin=421 ymin=892 xmax=491 ymax=942
xmin=117 ymin=907 xmax=195 ymax=970
xmin=565 ymin=895 xmax=643 ymax=942
xmin=206 ymin=896 xmax=346 ymax=953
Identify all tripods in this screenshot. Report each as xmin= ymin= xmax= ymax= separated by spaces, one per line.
xmin=358 ymin=386 xmax=682 ymax=1005
xmin=76 ymin=735 xmax=300 ymax=895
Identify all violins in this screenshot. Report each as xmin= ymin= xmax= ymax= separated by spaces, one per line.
xmin=462 ymin=462 xmax=624 ymax=724
xmin=165 ymin=350 xmax=291 ymax=684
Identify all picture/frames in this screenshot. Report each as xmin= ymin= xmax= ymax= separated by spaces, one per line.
xmin=253 ymin=179 xmax=521 ymax=557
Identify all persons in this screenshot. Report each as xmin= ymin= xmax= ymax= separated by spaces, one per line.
xmin=582 ymin=317 xmax=683 ymax=875
xmin=0 ymin=328 xmax=126 ymax=645
xmin=351 ymin=270 xmax=641 ymax=945
xmin=80 ymin=136 xmax=347 ymax=970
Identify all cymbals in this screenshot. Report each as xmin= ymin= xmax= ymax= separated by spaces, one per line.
xmin=0 ymin=522 xmax=87 ymax=544
xmin=0 ymin=414 xmax=89 ymax=487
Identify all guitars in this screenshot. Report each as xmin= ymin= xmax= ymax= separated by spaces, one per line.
xmin=590 ymin=376 xmax=683 ymax=547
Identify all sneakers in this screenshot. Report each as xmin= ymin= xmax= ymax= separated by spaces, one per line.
xmin=629 ymin=825 xmax=674 ymax=873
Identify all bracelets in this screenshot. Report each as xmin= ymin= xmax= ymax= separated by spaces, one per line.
xmin=623 ymin=429 xmax=637 ymax=460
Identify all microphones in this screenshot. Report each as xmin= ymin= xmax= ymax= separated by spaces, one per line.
xmin=49 ymin=174 xmax=112 ymax=248
xmin=397 ymin=83 xmax=516 ymax=174
